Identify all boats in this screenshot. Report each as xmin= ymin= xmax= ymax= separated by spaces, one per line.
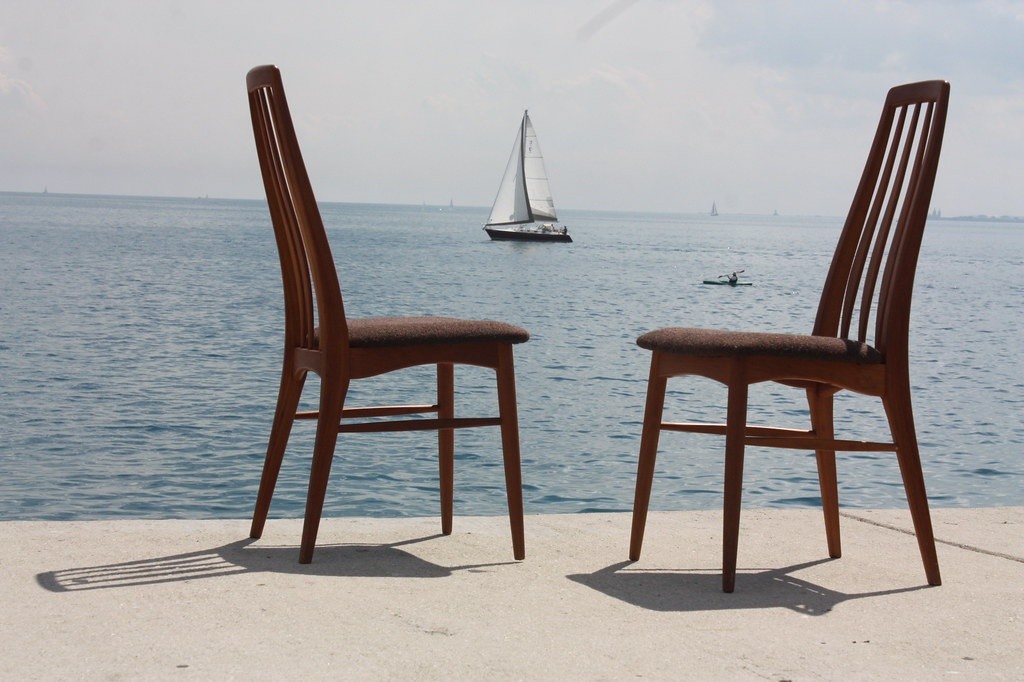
xmin=702 ymin=278 xmax=751 ymax=286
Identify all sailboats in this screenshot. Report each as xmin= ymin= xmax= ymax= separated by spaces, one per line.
xmin=709 ymin=201 xmax=718 ymax=216
xmin=481 ymin=109 xmax=573 ymax=242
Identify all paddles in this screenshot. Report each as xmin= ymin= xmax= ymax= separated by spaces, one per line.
xmin=718 ymin=270 xmax=744 ymax=278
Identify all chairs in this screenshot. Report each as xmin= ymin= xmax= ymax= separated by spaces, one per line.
xmin=624 ymin=79 xmax=952 ymax=595
xmin=243 ymin=63 xmax=525 ymax=560
